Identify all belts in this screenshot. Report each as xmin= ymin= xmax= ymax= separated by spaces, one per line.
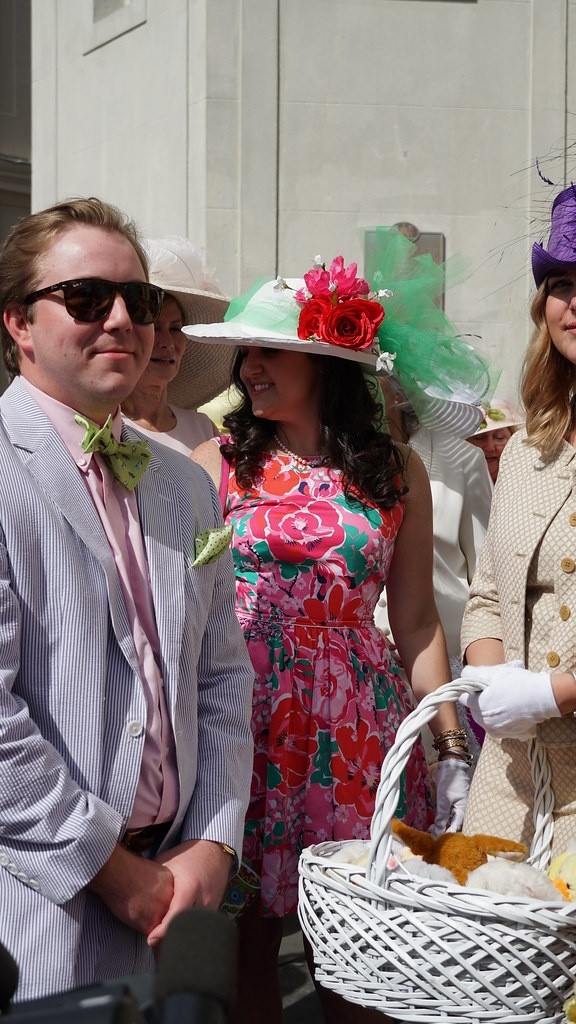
xmin=122 ymin=822 xmax=174 ymax=853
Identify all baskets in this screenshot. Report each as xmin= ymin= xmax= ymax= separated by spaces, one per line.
xmin=296 ymin=678 xmax=576 ymax=1024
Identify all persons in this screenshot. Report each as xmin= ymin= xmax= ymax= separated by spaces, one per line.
xmin=372 ymin=371 xmax=495 ymax=784
xmin=458 ymin=186 xmax=576 ymax=903
xmin=0 ymin=196 xmax=256 ymax=1024
xmin=464 ymin=396 xmax=523 ymax=488
xmin=116 ymin=284 xmax=241 ymax=458
xmin=181 ymin=275 xmax=472 ymax=1024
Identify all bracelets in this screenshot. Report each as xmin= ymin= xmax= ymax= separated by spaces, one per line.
xmin=218 ymin=843 xmax=235 ymax=855
xmin=565 ymin=669 xmax=576 ymax=718
xmin=433 ymin=727 xmax=473 ymax=767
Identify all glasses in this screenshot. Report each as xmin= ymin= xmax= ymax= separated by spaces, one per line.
xmin=23 ymin=278 xmax=165 ymax=325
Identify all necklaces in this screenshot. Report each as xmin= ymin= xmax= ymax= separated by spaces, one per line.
xmin=273 ymin=433 xmax=332 ymax=466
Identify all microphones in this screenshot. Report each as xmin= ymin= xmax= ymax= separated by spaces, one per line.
xmin=151 ymin=909 xmax=240 ymax=1024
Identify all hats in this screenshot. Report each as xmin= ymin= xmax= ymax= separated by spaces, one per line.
xmin=529 ymin=184 xmax=576 ymax=289
xmin=180 ymin=224 xmax=526 ymax=441
xmin=140 ymin=237 xmax=235 ymax=411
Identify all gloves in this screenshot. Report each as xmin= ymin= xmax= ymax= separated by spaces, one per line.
xmin=458 ymin=660 xmax=562 ymax=742
xmin=427 ymin=759 xmax=472 ymax=833
